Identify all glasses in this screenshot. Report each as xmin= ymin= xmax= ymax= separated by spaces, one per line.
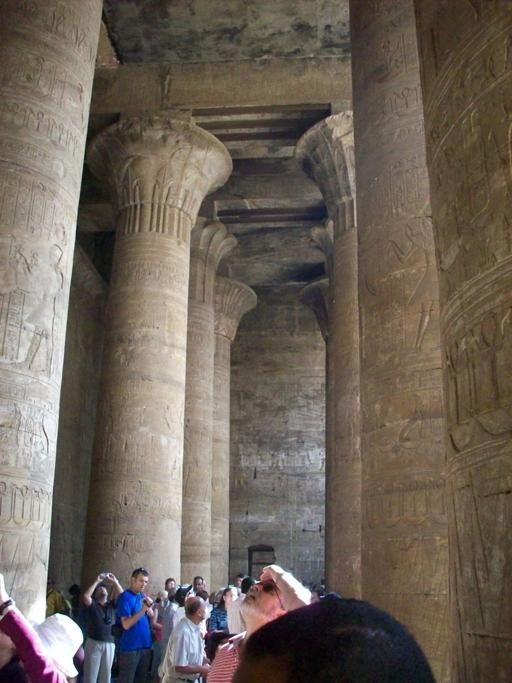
xmin=258 ymin=582 xmax=283 ymax=609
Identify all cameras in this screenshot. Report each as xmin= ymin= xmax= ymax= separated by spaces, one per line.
xmin=101 ymin=573 xmax=110 ymax=579
xmin=144 ymin=598 xmax=156 ymax=607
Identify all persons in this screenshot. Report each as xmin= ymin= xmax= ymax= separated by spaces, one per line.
xmin=1 ymin=564 xmax=436 ymax=682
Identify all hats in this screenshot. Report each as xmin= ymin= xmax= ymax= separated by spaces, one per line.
xmin=175 ymin=585 xmax=191 ymax=606
xmin=27 ymin=614 xmax=84 ymax=678
